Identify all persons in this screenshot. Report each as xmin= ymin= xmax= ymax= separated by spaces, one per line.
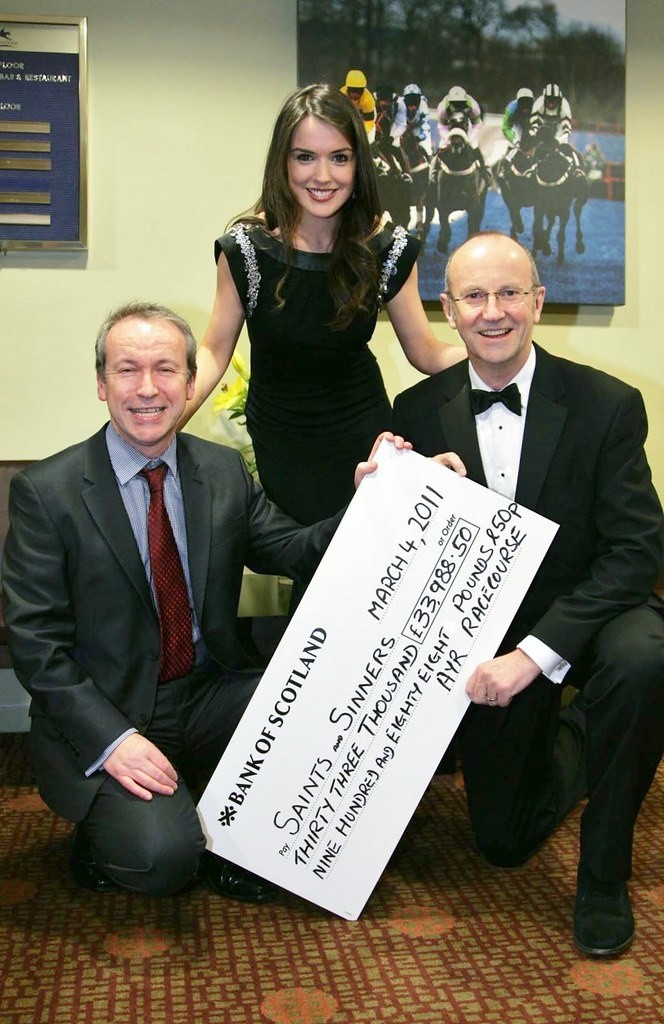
xmin=0 ymin=301 xmax=412 ymax=905
xmin=387 ymin=233 xmax=663 ymax=957
xmin=339 ymin=68 xmax=604 ymax=202
xmin=173 ymin=81 xmax=472 ymax=616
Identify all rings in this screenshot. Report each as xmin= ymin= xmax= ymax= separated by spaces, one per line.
xmin=486 ymin=696 xmax=497 ymax=701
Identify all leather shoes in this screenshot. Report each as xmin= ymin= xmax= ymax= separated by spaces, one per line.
xmin=67 ymin=828 xmax=120 ymax=892
xmin=207 ymin=856 xmax=278 ymax=905
xmin=576 ymin=865 xmax=635 ymax=955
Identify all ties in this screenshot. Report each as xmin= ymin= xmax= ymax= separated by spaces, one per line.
xmin=138 ymin=463 xmax=192 ymax=682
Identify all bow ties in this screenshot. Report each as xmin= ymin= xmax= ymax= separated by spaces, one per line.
xmin=468 ymin=383 xmax=521 ymax=416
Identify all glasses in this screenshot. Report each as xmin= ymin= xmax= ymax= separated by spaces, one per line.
xmin=446 ymin=286 xmax=538 ymax=309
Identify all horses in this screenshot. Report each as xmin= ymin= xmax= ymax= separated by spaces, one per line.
xmin=368 ymin=110 xmax=590 ymax=266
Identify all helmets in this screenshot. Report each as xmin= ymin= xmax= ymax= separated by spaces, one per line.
xmin=403 ymin=84 xmax=421 ymax=95
xmin=377 ymin=81 xmax=393 ymax=96
xmin=346 ymin=70 xmax=367 ymax=88
xmin=517 ymin=88 xmax=534 ymax=102
xmin=448 ymin=86 xmax=469 ymax=102
xmin=542 ymin=85 xmax=564 ymax=98
